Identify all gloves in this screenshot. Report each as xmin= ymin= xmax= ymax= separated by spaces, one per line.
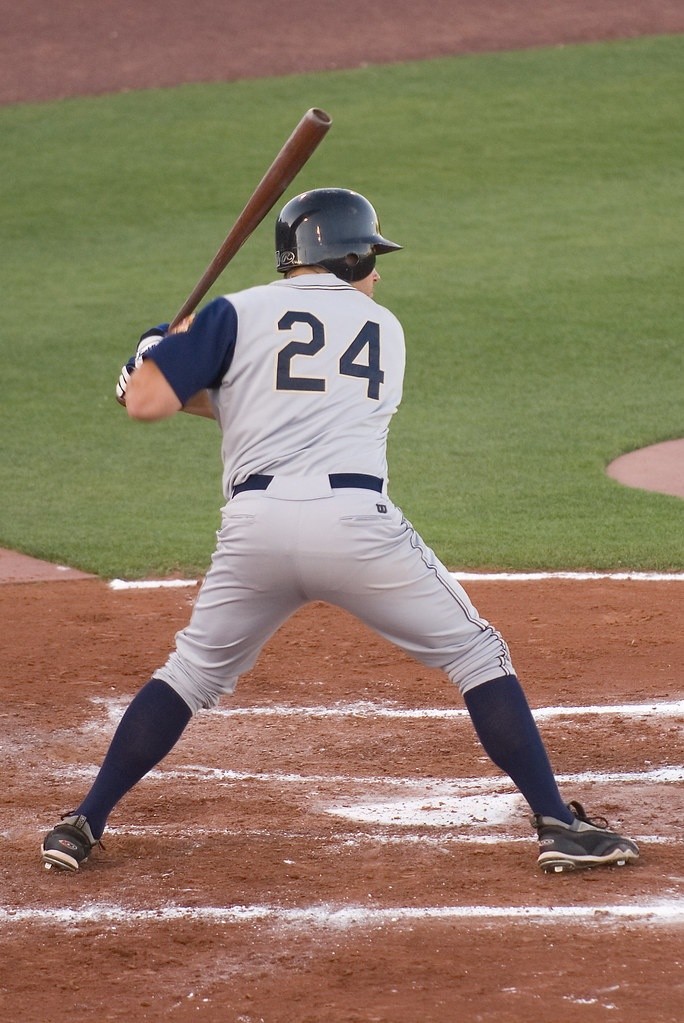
xmin=116 ymin=357 xmax=141 ymax=406
xmin=135 ymin=325 xmax=170 ymax=370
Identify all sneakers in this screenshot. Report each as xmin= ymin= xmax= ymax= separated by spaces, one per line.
xmin=40 ymin=811 xmax=106 ymax=871
xmin=529 ymin=800 xmax=642 ymax=873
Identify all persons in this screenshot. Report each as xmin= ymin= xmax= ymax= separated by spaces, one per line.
xmin=39 ymin=187 xmax=641 ymax=875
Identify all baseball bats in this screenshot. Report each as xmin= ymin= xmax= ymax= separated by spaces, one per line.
xmin=170 ymin=106 xmax=331 ymax=331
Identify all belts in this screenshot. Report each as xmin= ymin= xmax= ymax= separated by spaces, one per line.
xmin=232 ymin=472 xmax=384 ymax=499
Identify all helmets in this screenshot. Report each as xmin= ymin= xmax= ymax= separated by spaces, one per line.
xmin=274 ymin=188 xmax=404 ymax=283
